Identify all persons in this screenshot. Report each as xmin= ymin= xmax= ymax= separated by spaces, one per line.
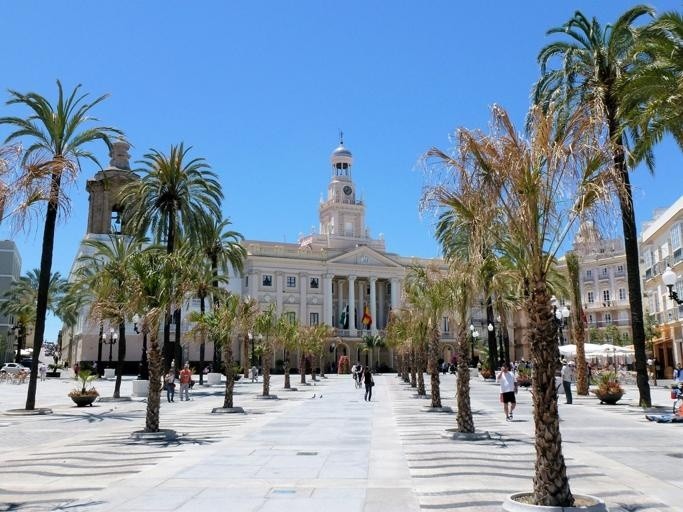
xmin=497 ymin=363 xmax=518 ymax=423
xmin=179 ymin=364 xmax=192 ymax=401
xmin=171 ymin=359 xmax=175 ymax=375
xmin=251 ymin=363 xmax=258 ymax=383
xmin=352 ymin=360 xmax=375 ymax=402
xmin=673 ymin=362 xmax=683 ymax=383
xmin=560 ymin=359 xmax=574 ymax=404
xmin=206 ymin=363 xmax=213 ymax=374
xmin=442 ymin=356 xmax=660 ymax=385
xmin=18 ymin=354 xmax=99 ymax=384
xmin=165 ymin=369 xmax=175 ymax=403
xmin=184 ymin=360 xmax=190 ymax=369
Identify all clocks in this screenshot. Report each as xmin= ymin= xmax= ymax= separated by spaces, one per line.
xmin=343 ymin=185 xmax=352 ymax=195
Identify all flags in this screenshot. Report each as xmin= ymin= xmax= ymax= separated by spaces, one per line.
xmin=361 ymin=302 xmax=372 ymax=327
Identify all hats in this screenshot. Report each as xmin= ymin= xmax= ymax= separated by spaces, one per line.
xmin=561 ymin=359 xmax=568 ymax=364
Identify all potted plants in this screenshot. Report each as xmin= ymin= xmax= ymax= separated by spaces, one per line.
xmin=516 ymin=366 xmax=532 ymax=388
xmin=589 ymin=369 xmax=626 ymax=404
xmin=233 ymin=362 xmax=242 ymax=381
xmin=480 ymin=347 xmax=492 ymax=379
xmin=67 ymin=367 xmax=99 ymax=408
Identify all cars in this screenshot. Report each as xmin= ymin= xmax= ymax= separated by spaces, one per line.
xmin=0 ymin=341 xmax=58 ymax=376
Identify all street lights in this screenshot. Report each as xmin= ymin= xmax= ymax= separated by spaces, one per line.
xmin=487 ymin=316 xmax=504 ymax=368
xmin=101 ymin=327 xmax=118 ymax=369
xmin=468 ymin=324 xmax=479 ymax=367
xmin=549 ymin=296 xmax=569 ymax=346
xmin=248 ymin=331 xmax=262 ymax=368
xmin=132 ymin=306 xmax=160 ymax=379
